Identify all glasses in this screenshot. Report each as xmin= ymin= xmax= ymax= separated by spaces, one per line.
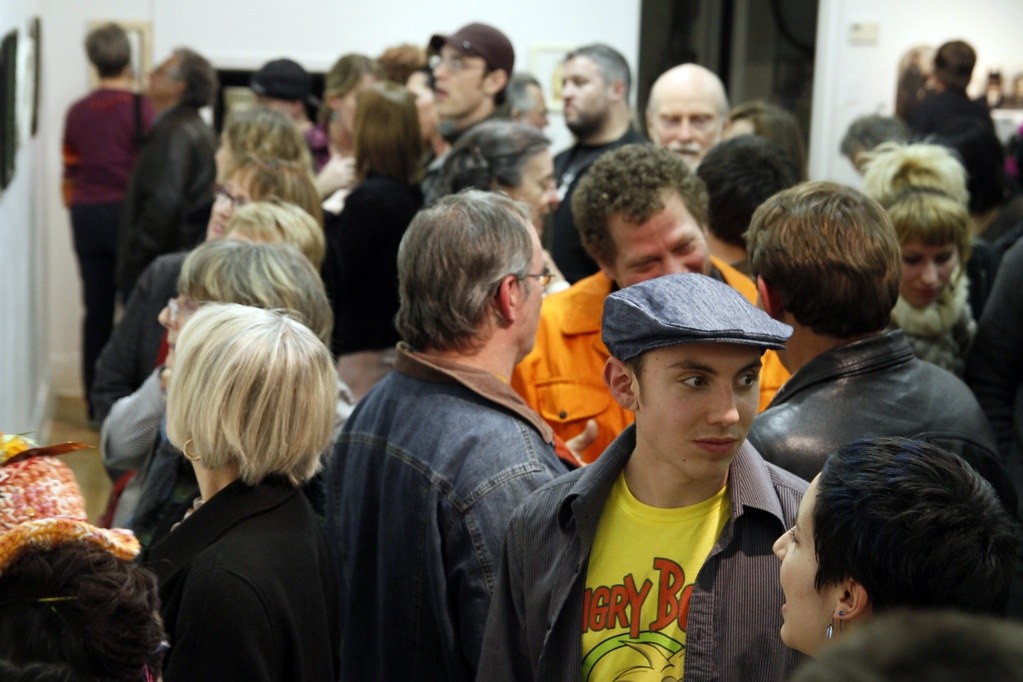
xmin=212 ymin=182 xmax=247 ymax=212
xmin=164 ymin=298 xmax=211 ymax=322
xmin=428 ymin=54 xmax=471 ymax=74
xmin=494 ymin=267 xmax=555 ymax=300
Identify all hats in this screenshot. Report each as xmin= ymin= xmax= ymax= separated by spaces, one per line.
xmin=428 ymin=23 xmax=515 ymax=77
xmin=250 ymin=59 xmax=323 ymax=109
xmin=1 ymin=432 xmax=141 ymax=567
xmin=601 ymin=273 xmax=794 ymax=362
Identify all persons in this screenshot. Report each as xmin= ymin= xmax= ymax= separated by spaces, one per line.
xmin=316 ymin=83 xmax=426 ymax=355
xmin=122 ymin=243 xmax=332 ymax=553
xmin=102 ymin=102 xmax=326 ymax=529
xmin=319 ymin=41 xmax=452 ymax=204
xmin=543 ymin=44 xmax=649 ymax=284
xmin=117 ymin=50 xmax=223 ymax=298
xmin=316 ymin=195 xmax=568 ymax=681
xmin=771 ymin=437 xmax=1022 ymax=682
xmin=745 ymin=180 xmax=1018 ymax=518
xmin=697 ymin=101 xmax=805 ymax=283
xmin=252 ymin=60 xmax=329 ymax=172
xmin=842 ymin=41 xmax=1023 ymax=508
xmin=150 ymin=301 xmax=339 ymax=681
xmin=511 ymin=145 xmax=790 ymax=469
xmin=0 ymin=435 xmax=168 ymax=682
xmin=427 ymin=119 xmax=555 ymax=236
xmin=433 ymin=24 xmax=548 ymax=142
xmin=475 ymin=274 xmax=812 ymax=682
xmin=646 ymin=63 xmax=729 ymax=170
xmin=61 ymin=24 xmax=145 ymax=418
